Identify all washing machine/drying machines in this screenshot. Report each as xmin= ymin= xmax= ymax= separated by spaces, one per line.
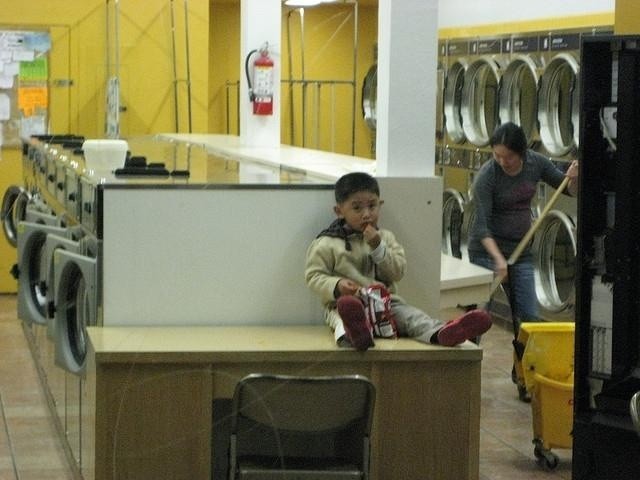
xmin=362 ymin=22 xmax=614 ymax=323
xmin=0 ymin=133 xmax=200 ymax=479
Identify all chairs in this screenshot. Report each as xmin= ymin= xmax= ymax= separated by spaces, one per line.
xmin=226 ymin=372 xmax=377 ymax=480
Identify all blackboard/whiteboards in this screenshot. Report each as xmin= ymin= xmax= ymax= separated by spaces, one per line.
xmin=1 ymin=24 xmax=50 ymax=149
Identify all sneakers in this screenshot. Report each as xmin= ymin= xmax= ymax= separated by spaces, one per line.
xmin=436 ymin=309 xmax=493 ymax=347
xmin=336 ymin=295 xmax=376 ymax=352
xmin=511 ymin=362 xmax=532 ymax=402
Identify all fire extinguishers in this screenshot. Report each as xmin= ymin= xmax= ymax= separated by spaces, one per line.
xmin=245 ymin=40 xmax=276 ymax=116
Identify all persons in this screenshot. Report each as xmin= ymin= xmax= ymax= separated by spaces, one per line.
xmin=465 ymin=117 xmax=578 ymax=404
xmin=305 ymin=170 xmax=495 ymax=350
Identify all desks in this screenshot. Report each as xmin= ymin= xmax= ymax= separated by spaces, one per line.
xmin=82 ymin=324 xmax=483 ymax=480
